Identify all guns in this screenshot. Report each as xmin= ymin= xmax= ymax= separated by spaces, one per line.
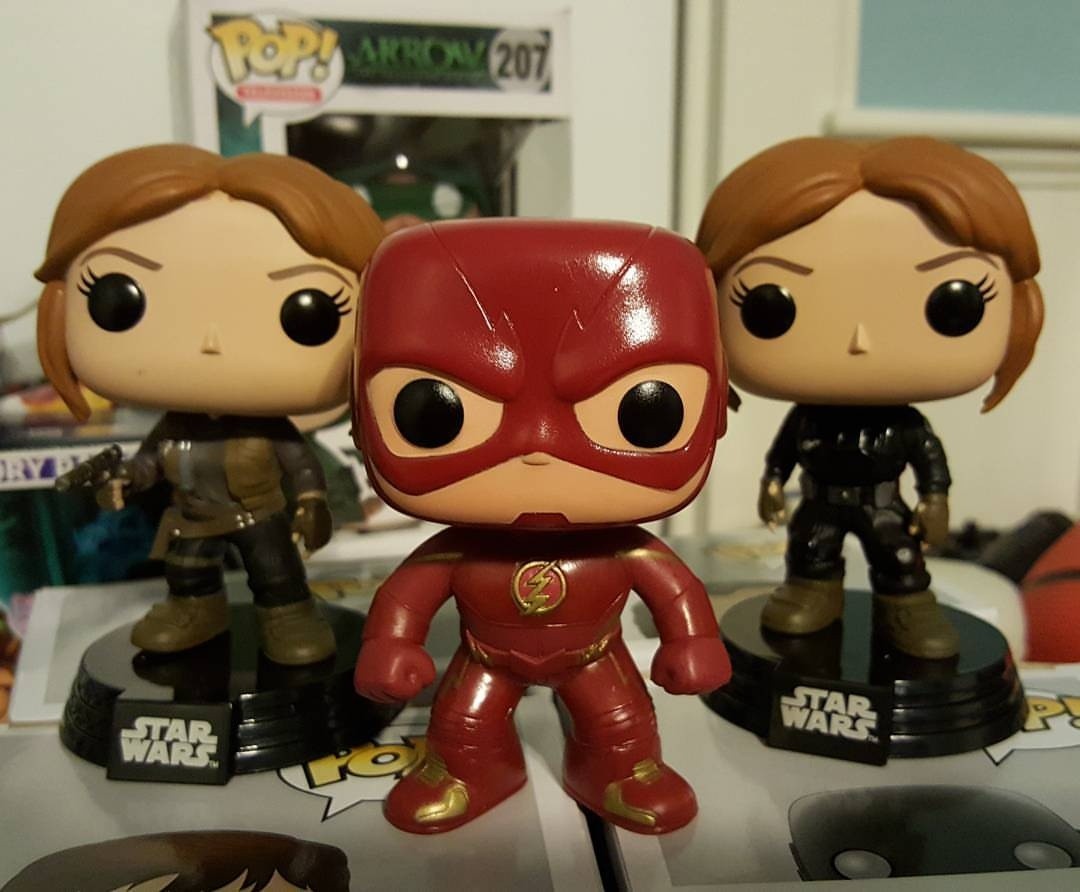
xmin=54 ymin=444 xmax=124 ymax=493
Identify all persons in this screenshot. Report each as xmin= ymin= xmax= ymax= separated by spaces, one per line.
xmin=699 ymin=138 xmax=1046 ymax=660
xmin=350 ymin=216 xmax=729 ymax=836
xmin=32 ymin=143 xmax=391 ymax=664
